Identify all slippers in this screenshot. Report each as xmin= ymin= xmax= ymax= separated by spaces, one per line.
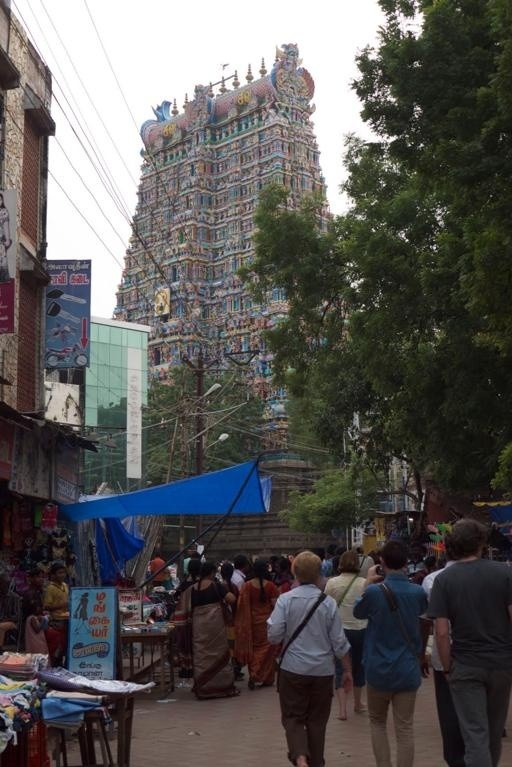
xmin=357 ymin=708 xmax=368 ymax=714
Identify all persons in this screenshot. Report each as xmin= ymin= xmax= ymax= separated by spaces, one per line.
xmin=423 ymin=520 xmax=512 ymax=766
xmin=265 ymin=551 xmax=353 ymax=767
xmin=352 ymin=540 xmax=428 ymax=767
xmin=151 ymin=546 xmax=439 ymax=697
xmin=23 ymin=563 xmax=70 ymax=669
xmin=323 ymin=551 xmax=368 ymax=721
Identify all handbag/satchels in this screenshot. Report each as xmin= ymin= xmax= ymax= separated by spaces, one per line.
xmin=223 ymin=603 xmax=232 ymax=617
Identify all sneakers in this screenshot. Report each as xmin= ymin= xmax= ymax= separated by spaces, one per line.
xmin=235 ymin=677 xmax=244 ymax=681
xmin=233 ymin=691 xmax=240 ymax=695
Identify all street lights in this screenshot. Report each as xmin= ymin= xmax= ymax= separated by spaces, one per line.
xmin=199 ymin=434 xmax=229 ymax=474
xmin=181 ymin=384 xmax=223 ymax=486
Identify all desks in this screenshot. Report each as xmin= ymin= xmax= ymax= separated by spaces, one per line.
xmin=50 ymin=694 xmax=134 ymax=767
xmin=119 ymin=631 xmax=174 ymax=700
